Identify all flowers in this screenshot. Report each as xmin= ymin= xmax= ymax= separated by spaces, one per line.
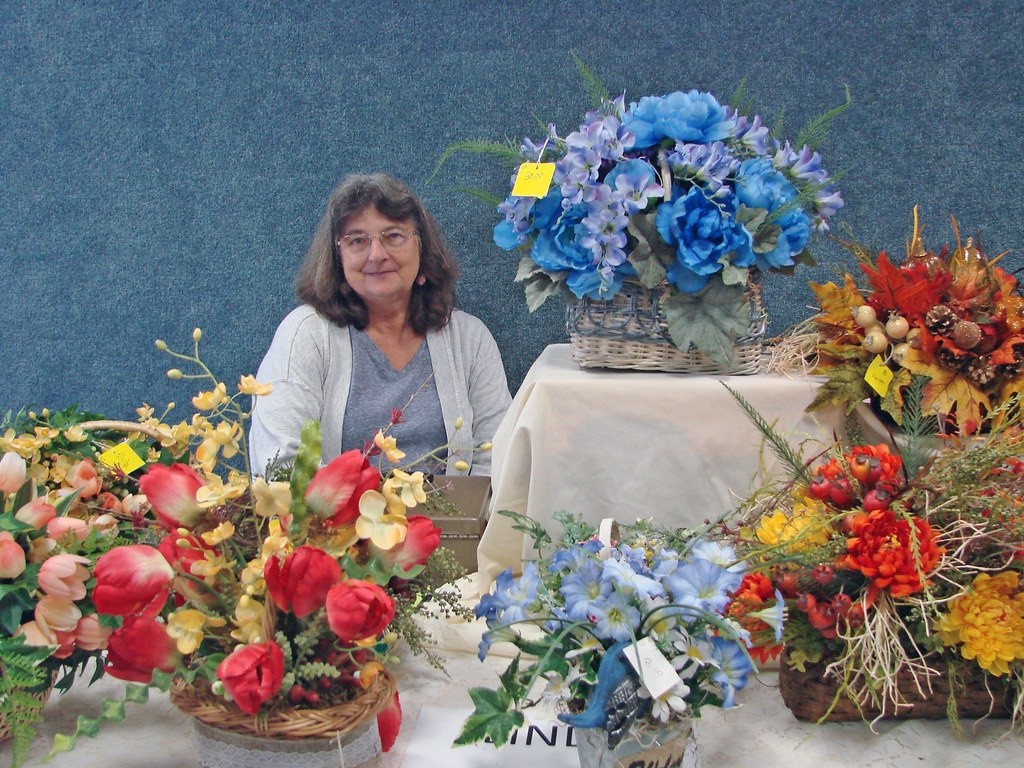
xmin=0 ymin=49 xmax=1024 ymax=768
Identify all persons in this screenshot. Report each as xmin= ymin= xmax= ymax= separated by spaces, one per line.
xmin=249 ymin=173 xmax=513 ymax=480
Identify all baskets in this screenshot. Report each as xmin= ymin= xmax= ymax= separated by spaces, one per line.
xmin=780 ymin=608 xmax=1013 ymax=722
xmin=171 ymin=669 xmax=397 ymax=740
xmin=0 ymin=662 xmax=60 ymax=742
xmin=567 ymin=266 xmax=766 ymax=377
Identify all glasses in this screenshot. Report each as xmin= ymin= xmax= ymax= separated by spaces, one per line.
xmin=334 ymin=228 xmax=422 ymax=256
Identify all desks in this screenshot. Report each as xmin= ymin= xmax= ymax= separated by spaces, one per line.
xmin=481 ymin=345 xmax=852 ymax=565
